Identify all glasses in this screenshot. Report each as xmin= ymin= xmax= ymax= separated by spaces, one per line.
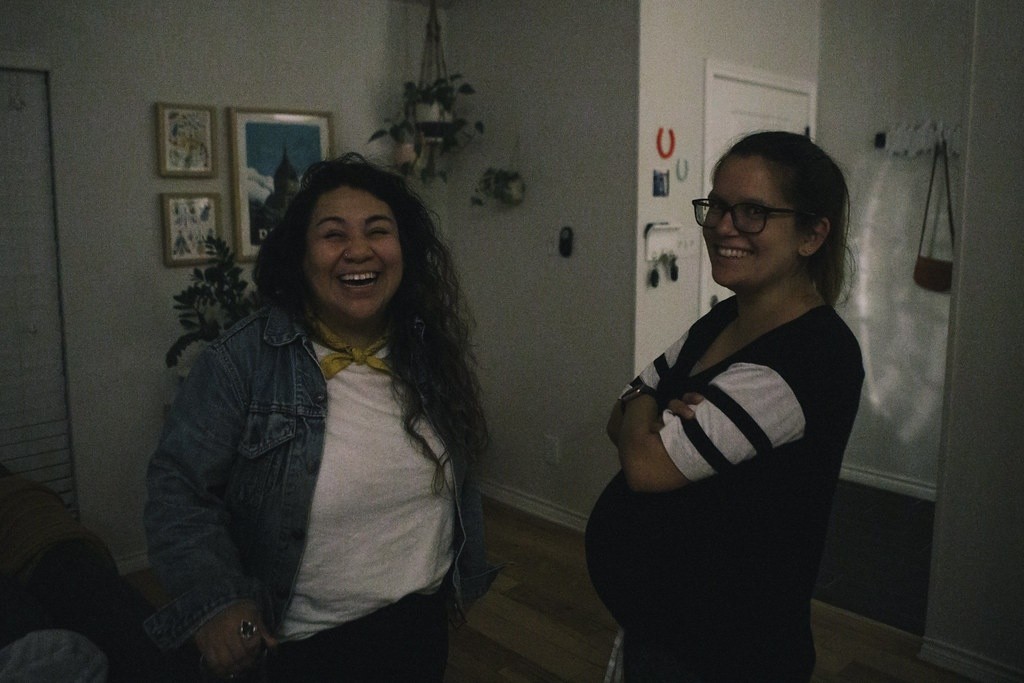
xmin=692 ymin=198 xmax=819 ymax=234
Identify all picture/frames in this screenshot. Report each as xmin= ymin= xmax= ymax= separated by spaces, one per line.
xmin=225 ymin=106 xmax=335 ymax=263
xmin=155 ymin=102 xmax=218 ymax=179
xmin=160 ymin=192 xmax=222 ymax=266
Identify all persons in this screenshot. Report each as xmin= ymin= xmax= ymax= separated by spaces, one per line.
xmin=142 ymin=155 xmax=493 ymax=683
xmin=584 ymin=130 xmax=866 ymax=683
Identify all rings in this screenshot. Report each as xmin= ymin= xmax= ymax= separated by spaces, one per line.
xmin=239 ymin=619 xmax=260 ymax=639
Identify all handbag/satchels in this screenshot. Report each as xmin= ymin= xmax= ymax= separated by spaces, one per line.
xmin=913 ymin=255 xmax=953 ymax=293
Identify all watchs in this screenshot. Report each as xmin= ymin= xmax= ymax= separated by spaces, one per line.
xmin=622 ymin=383 xmax=663 ymax=403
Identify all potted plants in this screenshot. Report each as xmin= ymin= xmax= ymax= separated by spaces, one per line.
xmin=470 ymin=166 xmax=527 ymax=206
xmin=367 ymin=0 xmax=485 ymax=184
